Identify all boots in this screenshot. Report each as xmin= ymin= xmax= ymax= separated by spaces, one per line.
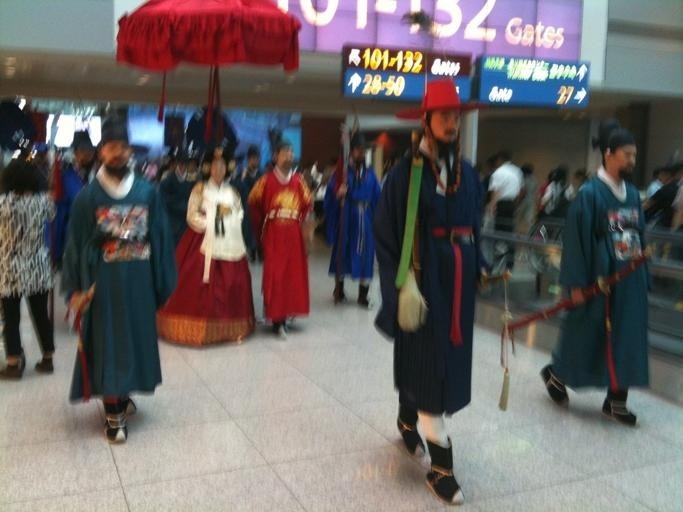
xmin=333 ymin=281 xmax=346 ymax=303
xmin=425 ymin=437 xmax=465 ymax=505
xmin=398 ymin=405 xmax=425 ymax=457
xmin=541 ymin=365 xmax=568 ymax=407
xmin=357 ymin=284 xmax=369 ymax=306
xmin=103 ymin=398 xmax=136 ymax=444
xmin=603 ymin=385 xmax=636 ymax=425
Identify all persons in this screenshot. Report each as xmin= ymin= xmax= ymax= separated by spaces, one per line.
xmin=472 ymin=150 xmax=588 ymax=301
xmin=539 ymin=122 xmax=649 ymax=428
xmin=640 ymin=153 xmax=682 ymax=266
xmin=306 ymin=132 xmax=414 ymax=306
xmin=0 ymin=117 xmax=314 ymax=445
xmin=372 ymin=79 xmax=488 ymax=505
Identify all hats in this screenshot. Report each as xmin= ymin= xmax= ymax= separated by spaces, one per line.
xmin=102 ymin=114 xmax=128 ymax=143
xmin=592 ymin=119 xmax=635 ymax=150
xmin=71 ymin=131 xmax=93 ymax=147
xmin=397 ymin=80 xmax=488 ymax=118
